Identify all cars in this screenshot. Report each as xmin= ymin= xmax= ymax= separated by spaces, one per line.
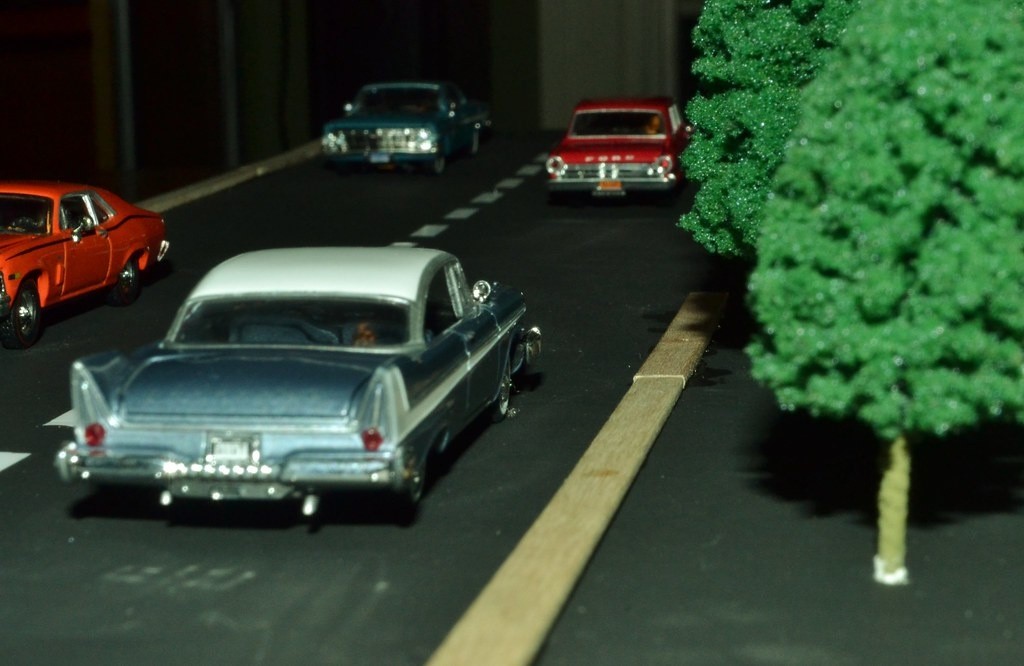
xmin=542 ymin=97 xmax=690 ymax=197
xmin=320 ymin=79 xmax=491 ymax=173
xmin=0 ymin=180 xmax=170 ymax=349
xmin=53 ymin=245 xmax=542 ymax=519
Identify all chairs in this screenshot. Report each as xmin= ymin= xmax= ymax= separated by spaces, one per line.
xmin=229 ymin=304 xmax=340 ymax=345
xmin=341 ymin=303 xmax=433 ymax=347
